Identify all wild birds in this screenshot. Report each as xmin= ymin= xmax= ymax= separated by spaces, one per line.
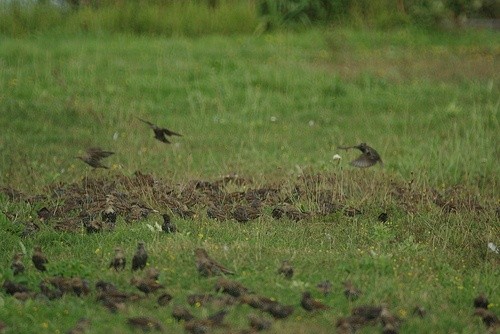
xmin=336 ymin=143 xmax=383 ymax=168
xmin=0 ymin=174 xmax=500 ymax=334
xmin=74 ymin=149 xmax=114 ymax=169
xmin=136 ymin=116 xmax=183 ymax=145
